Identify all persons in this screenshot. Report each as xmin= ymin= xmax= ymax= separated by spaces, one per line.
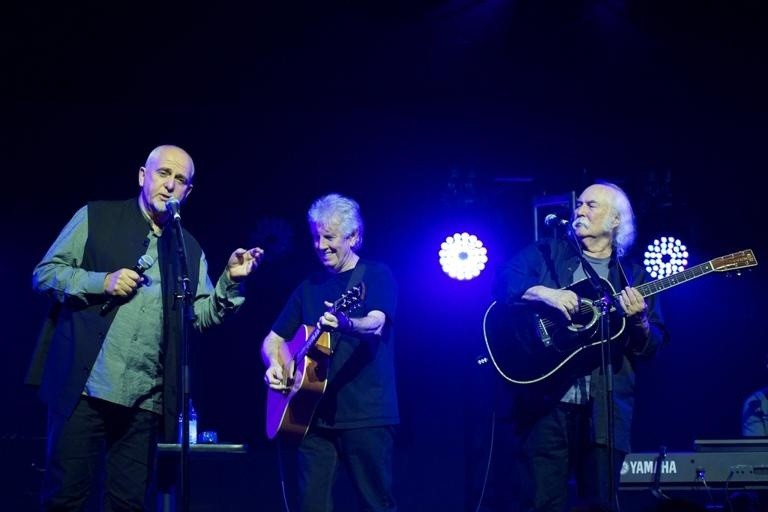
xmin=29 ymin=143 xmax=267 ymax=512
xmin=259 ymin=193 xmax=403 ymax=511
xmin=737 ymin=385 xmax=768 ymax=512
xmin=508 ymin=181 xmax=653 ymax=512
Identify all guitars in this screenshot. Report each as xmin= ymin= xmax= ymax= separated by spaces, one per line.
xmin=266 ymin=281 xmax=365 ymax=446
xmin=483 ymin=246 xmax=759 ymax=384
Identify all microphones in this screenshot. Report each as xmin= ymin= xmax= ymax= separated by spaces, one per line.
xmin=100 ymin=254 xmax=155 ymax=313
xmin=543 ymin=214 xmax=570 ymax=228
xmin=164 ymin=196 xmax=182 ymax=221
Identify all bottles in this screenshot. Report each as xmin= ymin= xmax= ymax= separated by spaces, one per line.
xmin=177 ymin=399 xmax=198 ymax=445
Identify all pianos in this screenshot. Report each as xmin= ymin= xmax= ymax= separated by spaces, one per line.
xmin=620 ymin=439 xmax=768 ymax=491
